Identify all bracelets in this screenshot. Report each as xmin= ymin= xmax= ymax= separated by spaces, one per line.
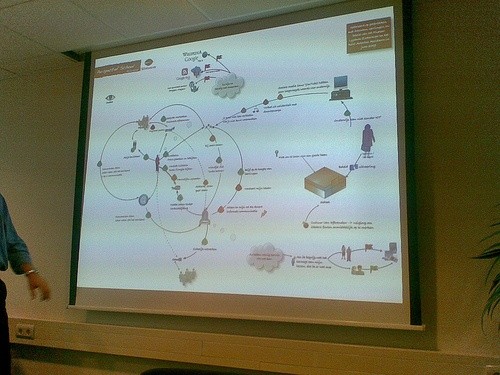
xmin=25 ymin=269 xmax=42 ymax=276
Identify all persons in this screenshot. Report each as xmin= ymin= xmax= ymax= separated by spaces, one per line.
xmin=0 ymin=193 xmax=51 ymax=375
